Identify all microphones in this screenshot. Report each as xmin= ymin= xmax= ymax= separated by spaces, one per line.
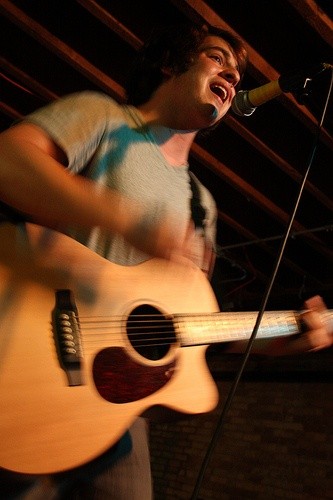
xmin=230 ymin=62 xmax=326 ymax=117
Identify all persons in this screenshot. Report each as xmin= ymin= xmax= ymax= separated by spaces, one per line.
xmin=1 ymin=23 xmax=333 ymax=500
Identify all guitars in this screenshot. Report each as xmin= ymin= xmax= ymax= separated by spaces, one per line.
xmin=0 ymin=223 xmax=332 ymax=472
